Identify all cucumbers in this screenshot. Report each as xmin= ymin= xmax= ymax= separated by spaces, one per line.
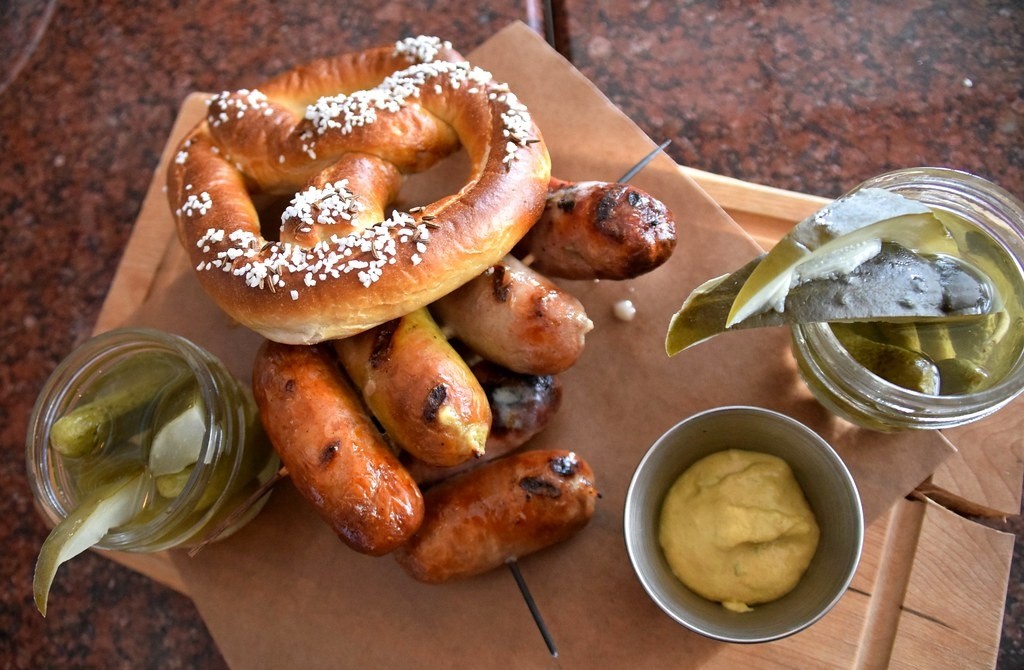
xmin=663 ymin=188 xmax=1003 ymax=395
xmin=29 ymin=352 xmax=208 ymax=619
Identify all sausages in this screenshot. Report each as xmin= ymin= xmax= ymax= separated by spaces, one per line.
xmin=252 ymin=176 xmax=678 ymax=585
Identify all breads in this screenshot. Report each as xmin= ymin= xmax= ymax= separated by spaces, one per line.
xmin=164 ymin=35 xmax=550 ymax=345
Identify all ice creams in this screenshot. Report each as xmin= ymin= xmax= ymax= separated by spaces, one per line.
xmin=662 ymin=450 xmax=821 ymax=616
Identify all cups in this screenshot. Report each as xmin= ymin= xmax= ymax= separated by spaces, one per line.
xmin=28 ymin=329 xmax=277 ymax=554
xmin=787 ymin=167 xmax=1024 ymax=433
xmin=621 ymin=406 xmax=864 ymax=647
